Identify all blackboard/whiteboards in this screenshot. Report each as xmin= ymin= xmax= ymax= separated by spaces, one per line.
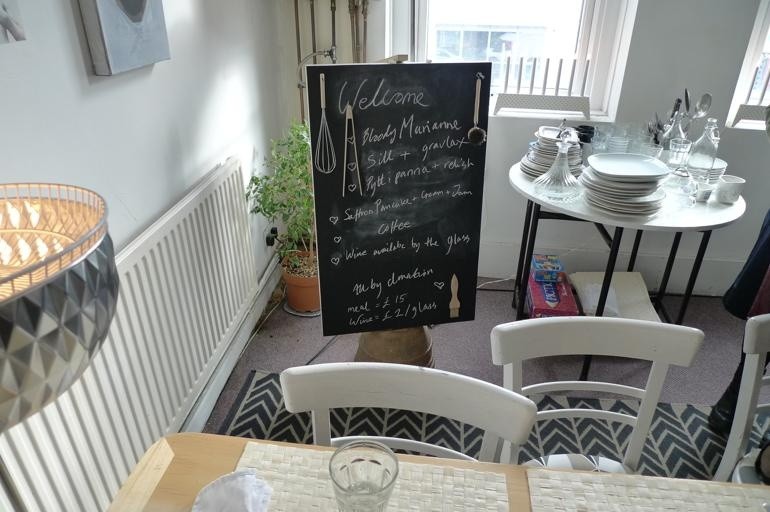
xmin=306 ymin=62 xmax=494 ymax=337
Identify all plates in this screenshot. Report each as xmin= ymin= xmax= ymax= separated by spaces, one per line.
xmin=520 ymin=126 xmax=586 ymax=180
xmin=577 ymin=152 xmax=673 ymax=218
xmin=710 ymin=158 xmax=730 ymax=183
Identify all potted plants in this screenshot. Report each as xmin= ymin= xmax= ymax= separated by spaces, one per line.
xmin=243 ymin=123 xmax=321 ymax=320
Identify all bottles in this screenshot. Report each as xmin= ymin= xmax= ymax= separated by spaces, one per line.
xmin=686 ymin=118 xmax=720 ymax=185
xmin=659 ymin=112 xmax=685 ymax=163
xmin=531 ymin=128 xmax=584 ymax=204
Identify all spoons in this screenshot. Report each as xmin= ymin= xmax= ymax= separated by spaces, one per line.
xmin=685 ymin=88 xmax=691 ymax=114
xmin=683 ymin=92 xmax=712 ymax=131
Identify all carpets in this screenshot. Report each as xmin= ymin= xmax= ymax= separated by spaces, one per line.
xmin=220 ymin=368 xmax=768 ymax=484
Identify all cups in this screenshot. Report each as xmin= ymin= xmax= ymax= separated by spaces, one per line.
xmin=640 ymin=143 xmax=663 ymax=158
xmin=591 ymin=122 xmax=640 ymax=152
xmin=329 ymin=440 xmax=399 ymax=512
xmin=669 ymin=138 xmax=692 ymax=164
xmin=715 ymin=174 xmax=746 ymax=204
xmin=696 ymin=182 xmax=714 ymax=203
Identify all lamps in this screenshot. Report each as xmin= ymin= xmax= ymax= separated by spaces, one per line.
xmin=0 ymin=179 xmax=119 ymax=512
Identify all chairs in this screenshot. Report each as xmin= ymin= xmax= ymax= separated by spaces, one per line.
xmin=280 ymin=361 xmax=535 ymax=464
xmin=712 ymin=315 xmax=769 ymax=486
xmin=491 ymin=314 xmax=705 ymax=476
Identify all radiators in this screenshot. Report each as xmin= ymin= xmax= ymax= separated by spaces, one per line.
xmin=0 ymin=154 xmax=260 ymax=512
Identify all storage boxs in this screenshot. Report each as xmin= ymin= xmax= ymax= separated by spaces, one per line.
xmin=525 ymin=273 xmax=579 ymax=318
xmin=530 ymin=252 xmax=566 ymax=282
